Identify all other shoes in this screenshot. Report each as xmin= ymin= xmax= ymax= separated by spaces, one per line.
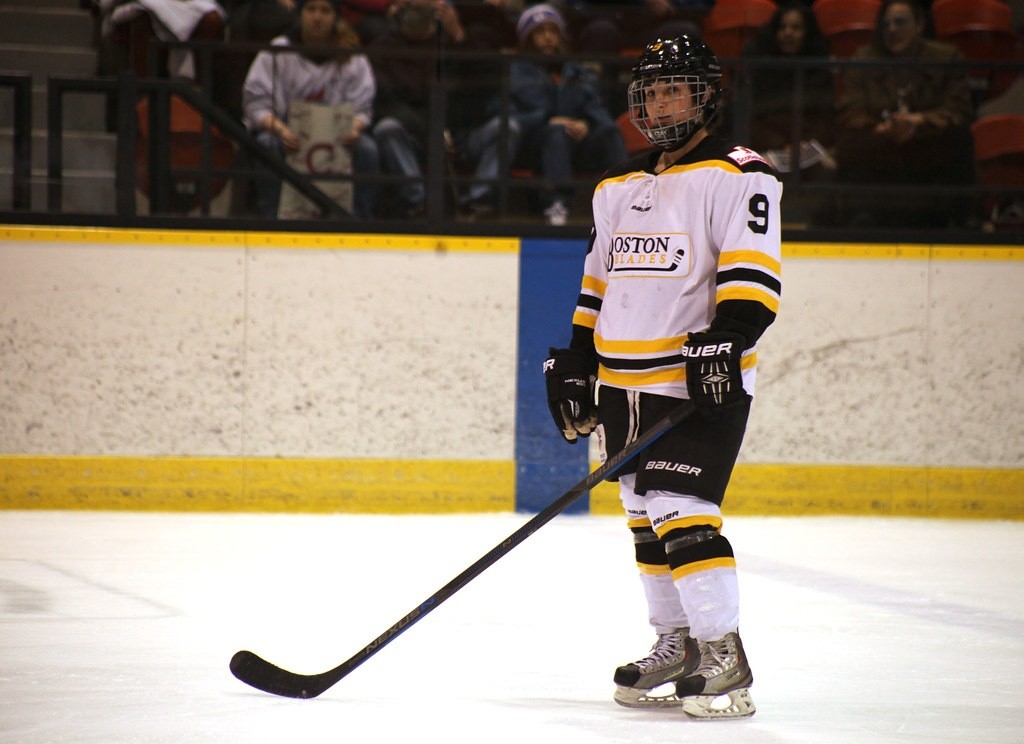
xmin=544 ymin=201 xmax=568 ymax=226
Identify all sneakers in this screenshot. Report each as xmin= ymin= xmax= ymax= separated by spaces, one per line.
xmin=614 ymin=626 xmax=701 ymax=708
xmin=676 ymin=627 xmax=756 ymax=720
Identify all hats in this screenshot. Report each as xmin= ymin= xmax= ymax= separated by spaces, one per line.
xmin=517 ymin=5 xmax=579 ymax=58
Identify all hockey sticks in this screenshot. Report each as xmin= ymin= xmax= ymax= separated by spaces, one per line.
xmin=229 ymin=398 xmax=701 ymax=699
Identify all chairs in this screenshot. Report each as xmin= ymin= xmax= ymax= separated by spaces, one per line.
xmin=135 ymin=1 xmax=1024 ymax=228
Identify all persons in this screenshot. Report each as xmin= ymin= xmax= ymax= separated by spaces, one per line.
xmin=240 ymin=0 xmax=628 ymax=227
xmin=542 ymin=34 xmax=787 ymax=723
xmin=724 ymin=0 xmax=979 ymax=228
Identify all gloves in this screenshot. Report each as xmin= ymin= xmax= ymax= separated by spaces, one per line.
xmin=542 ymin=348 xmax=601 ymax=444
xmin=684 ymin=314 xmax=748 ymax=408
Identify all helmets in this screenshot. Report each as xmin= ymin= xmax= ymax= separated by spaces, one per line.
xmin=628 ymin=32 xmax=722 ymax=144
